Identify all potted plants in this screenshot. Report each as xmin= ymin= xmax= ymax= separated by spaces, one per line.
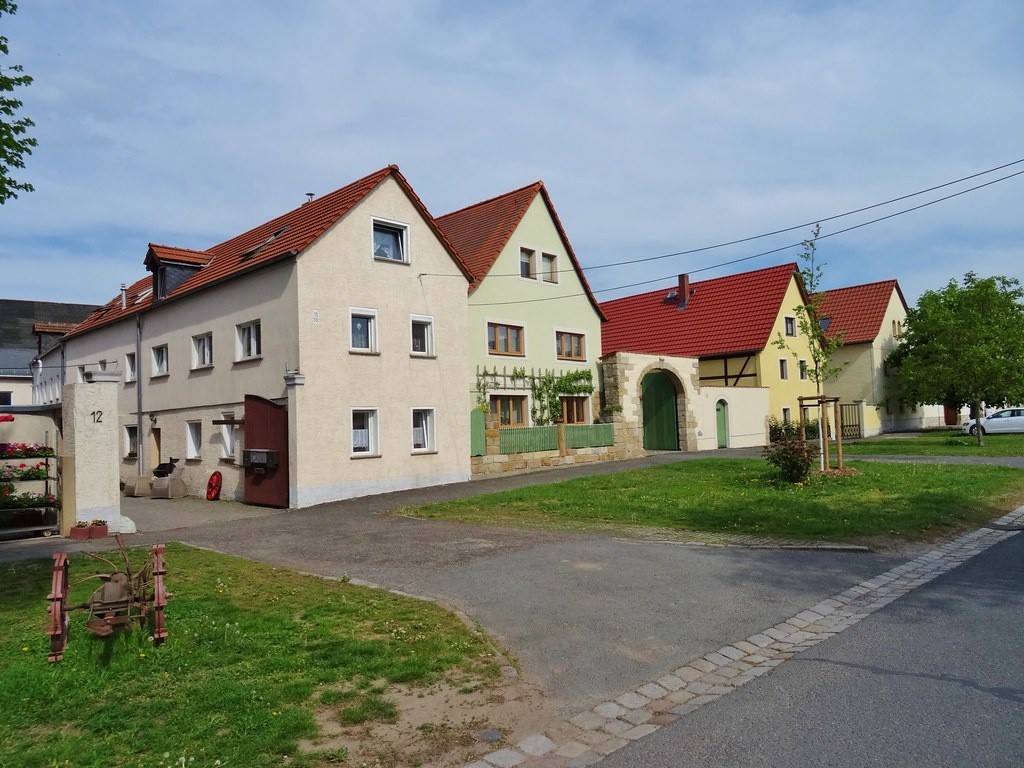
xmin=128 ymin=450 xmax=136 ymax=456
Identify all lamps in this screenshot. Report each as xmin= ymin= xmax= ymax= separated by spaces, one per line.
xmin=149 ymin=412 xmax=157 ymax=424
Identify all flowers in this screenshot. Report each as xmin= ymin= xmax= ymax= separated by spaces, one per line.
xmin=91 ymin=520 xmax=108 ymax=526
xmin=0 ymin=483 xmax=60 ymax=508
xmin=0 ymin=461 xmax=50 ymax=480
xmin=77 ymin=521 xmax=88 ymax=528
xmin=0 ymin=442 xmax=55 ymax=457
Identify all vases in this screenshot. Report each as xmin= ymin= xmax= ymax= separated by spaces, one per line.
xmin=0 ymin=478 xmax=11 ymax=482
xmin=0 ymin=505 xmax=12 ymax=509
xmin=89 ymin=525 xmax=108 ymax=538
xmin=20 ymin=477 xmax=29 ymax=480
xmin=70 ymin=526 xmax=89 ymax=539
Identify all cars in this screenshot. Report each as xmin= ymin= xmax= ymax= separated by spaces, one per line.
xmin=962 ymin=407 xmax=1024 ymax=437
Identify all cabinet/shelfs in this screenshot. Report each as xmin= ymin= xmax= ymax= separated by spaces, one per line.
xmin=0 ymin=429 xmax=61 ymax=537
